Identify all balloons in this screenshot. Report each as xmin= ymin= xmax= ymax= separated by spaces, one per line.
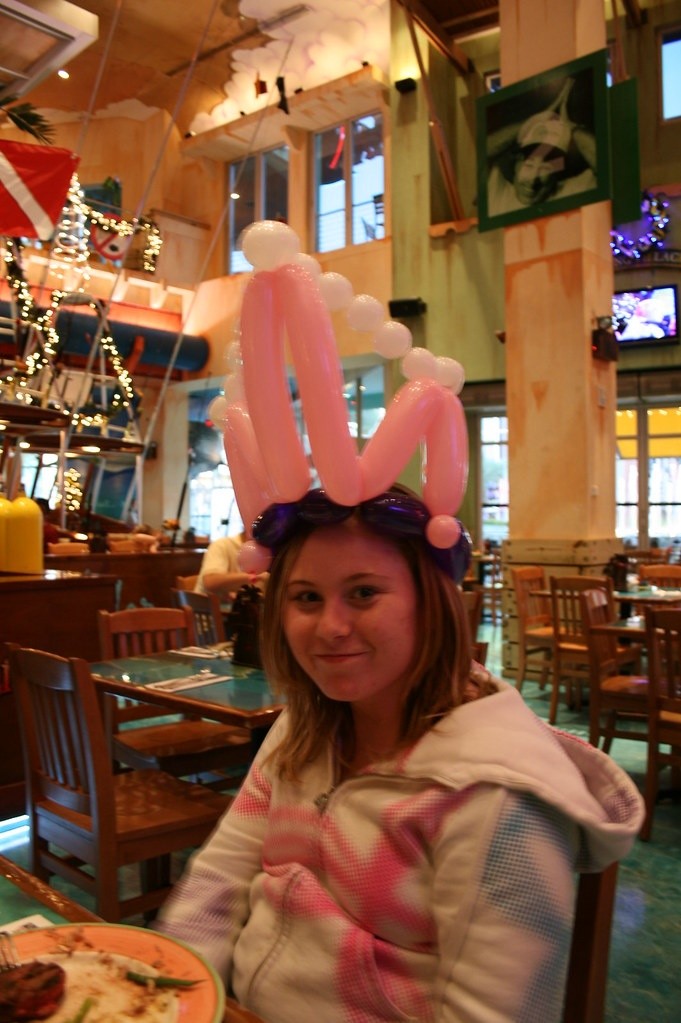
xmin=209 ymin=221 xmax=475 ymax=581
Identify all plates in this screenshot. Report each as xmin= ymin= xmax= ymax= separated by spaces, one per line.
xmin=0 ymin=922 xmax=226 ymax=1023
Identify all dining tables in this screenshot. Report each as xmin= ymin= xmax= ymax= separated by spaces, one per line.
xmin=0 ymin=857 xmax=261 ymax=1023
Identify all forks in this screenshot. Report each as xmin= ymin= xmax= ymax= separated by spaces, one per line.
xmin=0 ymin=929 xmax=21 ymax=972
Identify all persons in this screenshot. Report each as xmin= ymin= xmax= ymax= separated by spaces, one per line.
xmin=193 ymin=528 xmax=271 ymax=647
xmin=486 ymin=118 xmax=599 ymax=218
xmin=152 ymin=479 xmax=647 ymax=1023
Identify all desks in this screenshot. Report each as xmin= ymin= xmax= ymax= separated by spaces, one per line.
xmin=611 ymin=588 xmax=681 ymax=611
xmin=89 ymin=653 xmax=290 ymax=774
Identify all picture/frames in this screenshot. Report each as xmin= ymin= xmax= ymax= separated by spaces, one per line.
xmin=474 ymin=47 xmax=609 ymax=235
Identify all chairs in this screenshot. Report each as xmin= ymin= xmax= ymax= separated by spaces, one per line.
xmin=0 ymin=536 xmax=681 ymax=1023
